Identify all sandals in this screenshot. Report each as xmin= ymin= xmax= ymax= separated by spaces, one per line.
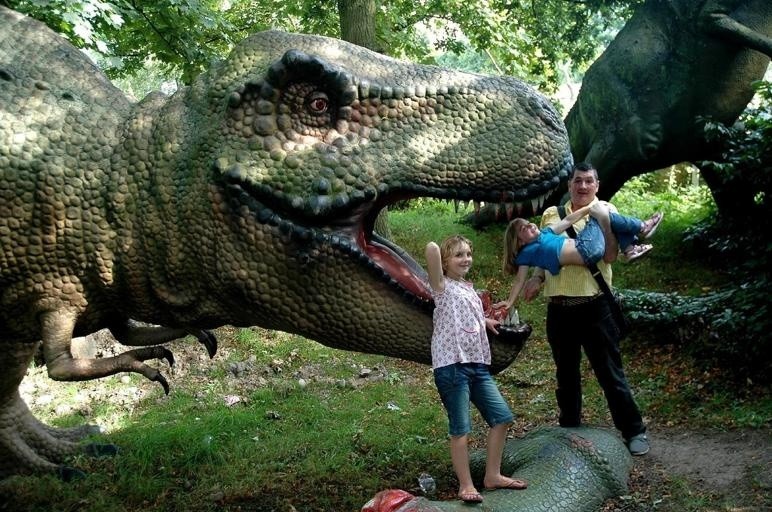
xmin=623 ymin=243 xmax=654 ymax=264
xmin=640 ymin=211 xmax=664 ymax=240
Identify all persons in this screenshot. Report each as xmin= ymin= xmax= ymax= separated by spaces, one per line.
xmin=493 ymin=199 xmax=665 ymax=310
xmin=524 ymin=162 xmax=649 ymax=457
xmin=424 ymin=233 xmax=528 ymax=502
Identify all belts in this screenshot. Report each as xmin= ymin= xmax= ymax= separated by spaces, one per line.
xmin=553 ymin=293 xmax=601 ymax=307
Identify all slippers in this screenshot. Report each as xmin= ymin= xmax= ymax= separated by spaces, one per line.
xmin=483 ymin=474 xmax=528 ymax=491
xmin=456 ymin=488 xmax=485 ymax=503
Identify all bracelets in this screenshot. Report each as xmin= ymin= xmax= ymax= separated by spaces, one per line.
xmin=532 ymin=274 xmax=544 ymax=282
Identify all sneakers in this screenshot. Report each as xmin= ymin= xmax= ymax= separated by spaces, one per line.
xmin=628 ymin=430 xmax=650 ymax=457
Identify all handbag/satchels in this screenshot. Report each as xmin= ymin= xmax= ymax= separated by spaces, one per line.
xmin=595 ymin=287 xmax=632 ymax=346
